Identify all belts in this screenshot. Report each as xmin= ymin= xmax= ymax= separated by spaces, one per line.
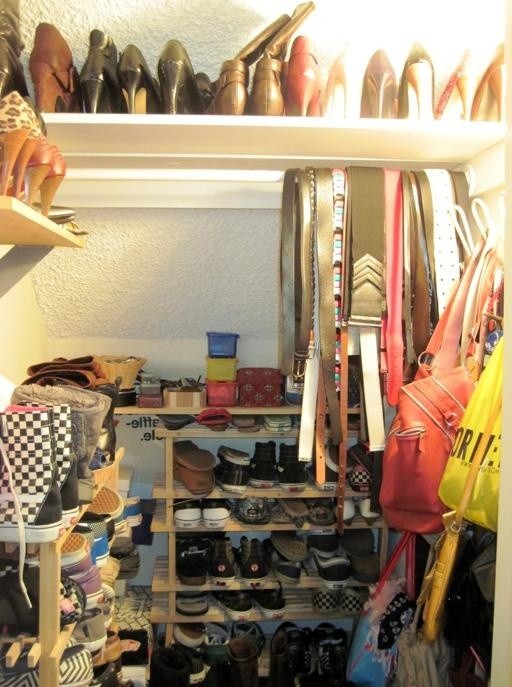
xmin=278 ymin=165 xmax=472 ymax=534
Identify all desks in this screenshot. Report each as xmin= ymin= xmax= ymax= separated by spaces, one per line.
xmin=0 ymin=441 xmax=127 ymax=687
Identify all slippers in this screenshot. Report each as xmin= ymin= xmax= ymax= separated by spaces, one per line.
xmin=157 ymin=412 xmax=292 ymax=434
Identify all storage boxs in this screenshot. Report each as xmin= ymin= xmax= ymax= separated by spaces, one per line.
xmin=107 ymin=583 xmax=154 ymax=666
xmin=163 ymin=331 xmax=283 ymax=408
xmin=111 ymin=664 xmax=148 ymax=687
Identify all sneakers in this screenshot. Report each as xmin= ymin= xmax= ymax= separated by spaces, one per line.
xmin=0 ymin=488 xmax=145 ymax=687
xmin=247 ymin=440 xmax=277 ymax=488
xmin=173 ymin=498 xmax=201 ymax=527
xmin=213 ymin=445 xmax=250 ymax=471
xmin=235 ymin=498 xmax=272 ymax=525
xmin=151 ymin=530 xmax=346 ymax=687
xmin=173 ymin=439 xmax=216 ymax=472
xmin=173 ymin=463 xmax=213 ymax=493
xmin=211 ymin=462 xmax=250 ymax=494
xmin=202 ymin=499 xmax=231 ymax=530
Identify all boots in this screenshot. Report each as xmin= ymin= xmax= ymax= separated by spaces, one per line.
xmin=249 ymin=1 xmax=315 ymax=116
xmin=0 ymin=1 xmax=30 ymax=98
xmin=0 ymin=355 xmax=124 ymax=544
xmin=205 ymin=0 xmax=289 ymax=115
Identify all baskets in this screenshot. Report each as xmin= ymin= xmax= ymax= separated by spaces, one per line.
xmin=91 ymin=355 xmax=147 ymax=390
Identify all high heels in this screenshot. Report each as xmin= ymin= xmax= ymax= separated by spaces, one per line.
xmin=359 ymin=47 xmax=395 ymax=119
xmin=320 ymin=42 xmax=358 ymax=118
xmin=434 ymin=46 xmax=472 ymax=119
xmin=396 ymin=40 xmax=434 ymax=118
xmin=156 ymin=40 xmax=204 ymax=115
xmin=75 ymin=26 xmax=129 ymax=113
xmin=116 ymin=44 xmax=163 ymax=114
xmin=0 ymin=92 xmax=89 ymax=237
xmin=285 ymin=35 xmax=319 ymax=116
xmin=28 ymin=23 xmax=83 ymax=113
xmin=469 ymin=34 xmax=505 ymax=121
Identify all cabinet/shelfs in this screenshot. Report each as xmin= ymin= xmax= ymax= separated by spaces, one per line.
xmin=148 ymin=426 xmax=397 ymax=687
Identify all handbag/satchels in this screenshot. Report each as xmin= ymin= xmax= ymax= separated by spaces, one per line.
xmin=390 ymin=243 xmax=509 ymax=537
xmin=346 ymin=527 xmax=479 ymax=687
